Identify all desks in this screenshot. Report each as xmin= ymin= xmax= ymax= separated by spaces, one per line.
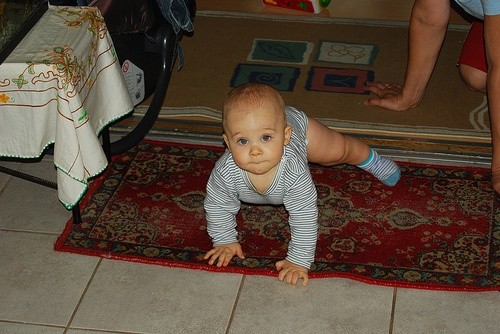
xmin=0 ymin=5 xmax=135 ymax=225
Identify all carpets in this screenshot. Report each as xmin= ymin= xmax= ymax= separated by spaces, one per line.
xmin=132 ymin=9 xmax=492 ymax=140
xmin=54 ymin=139 xmax=500 ymax=293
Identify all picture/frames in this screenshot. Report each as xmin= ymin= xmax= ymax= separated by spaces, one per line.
xmin=0 ymin=0 xmax=49 ymax=65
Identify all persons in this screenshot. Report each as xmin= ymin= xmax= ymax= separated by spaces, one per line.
xmin=361 ymin=0 xmax=500 ymax=197
xmin=204 ymin=81 xmax=402 ymax=287
